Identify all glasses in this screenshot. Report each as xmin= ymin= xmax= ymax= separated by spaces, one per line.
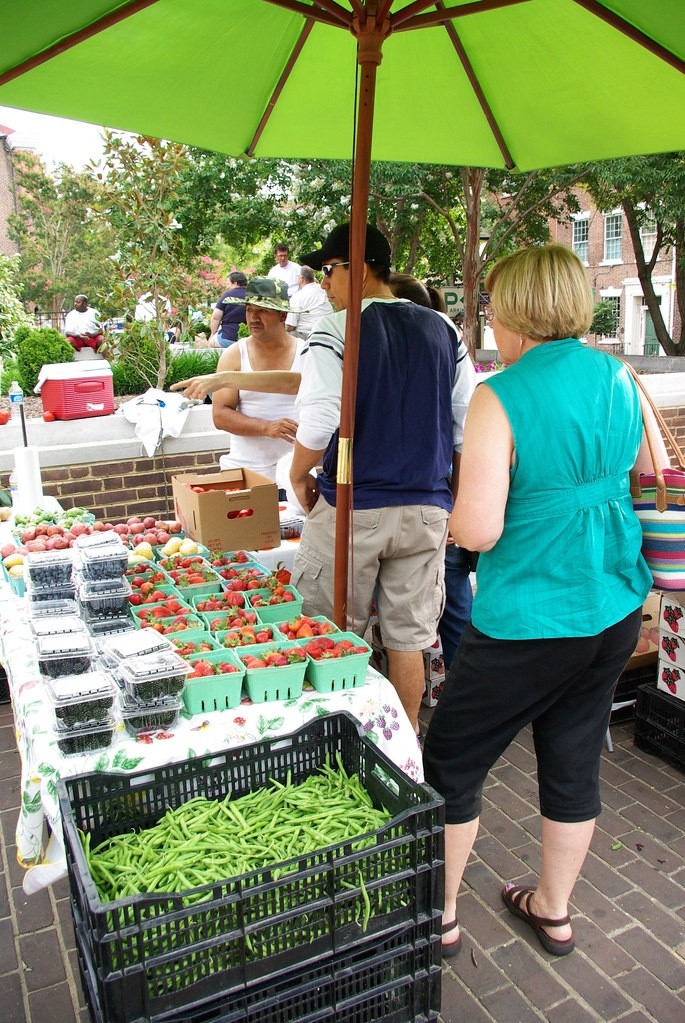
xmin=322 ymin=258 xmax=376 ymax=277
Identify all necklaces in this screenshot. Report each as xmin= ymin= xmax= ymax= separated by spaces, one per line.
xmin=366 ymin=294 xmax=399 ymax=299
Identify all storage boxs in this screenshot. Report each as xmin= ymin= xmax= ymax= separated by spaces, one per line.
xmin=55 ymin=710 xmax=447 ymax=1023
xmin=631 ymin=591 xmax=661 ymax=658
xmin=372 ymin=610 xmax=444 ymax=653
xmin=657 ymin=591 xmax=685 ymax=701
xmin=370 ymin=642 xmax=447 ymax=680
xmin=32 ymin=359 xmax=115 ymax=421
xmin=153 ymin=916 xmax=443 ymax=1023
xmin=0 ymin=505 xmax=374 ymax=716
xmin=170 ymin=466 xmax=281 ymax=552
xmin=373 ymin=659 xmax=446 ymax=708
xmin=634 ymin=681 xmax=685 ymax=776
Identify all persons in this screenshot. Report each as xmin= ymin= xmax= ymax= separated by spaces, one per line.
xmin=422 ymin=243 xmax=671 ymax=959
xmin=290 ymin=224 xmax=458 ymax=738
xmin=169 ymin=273 xmax=479 ymax=675
xmin=135 ymin=291 xmax=181 ymax=344
xmin=205 ymin=273 xmax=248 ymax=349
xmin=268 ymin=244 xmax=302 ymax=298
xmin=64 ymin=294 xmax=104 ymax=353
xmin=285 ymin=265 xmax=334 ymax=341
xmin=211 ymin=277 xmax=305 ymax=503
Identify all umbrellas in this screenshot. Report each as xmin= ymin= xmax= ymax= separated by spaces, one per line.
xmin=0 ymin=0 xmax=685 ymax=633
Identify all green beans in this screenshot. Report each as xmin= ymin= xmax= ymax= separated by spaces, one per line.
xmin=74 ymin=746 xmax=416 ymax=998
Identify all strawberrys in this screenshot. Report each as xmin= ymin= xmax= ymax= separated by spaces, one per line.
xmin=125 ymin=550 xmax=368 ymax=680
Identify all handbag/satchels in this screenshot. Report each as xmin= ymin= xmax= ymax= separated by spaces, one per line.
xmin=609 ymin=352 xmax=685 ymax=591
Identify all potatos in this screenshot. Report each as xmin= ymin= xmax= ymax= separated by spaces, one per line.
xmin=0 ymin=517 xmax=201 ymax=585
xmin=0 ymin=510 xmax=11 ymax=520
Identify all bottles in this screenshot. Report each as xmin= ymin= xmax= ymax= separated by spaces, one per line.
xmin=8 ymin=381 xmax=24 ymax=423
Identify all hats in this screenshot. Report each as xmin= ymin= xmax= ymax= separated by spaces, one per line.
xmin=222 ymin=276 xmax=310 ymax=313
xmin=299 ymin=223 xmax=391 ymax=271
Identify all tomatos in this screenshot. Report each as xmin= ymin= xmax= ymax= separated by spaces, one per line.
xmin=184 ymin=483 xmax=254 ymax=519
xmin=42 ymin=411 xmax=56 ymax=422
xmin=0 ymin=409 xmax=9 ymax=425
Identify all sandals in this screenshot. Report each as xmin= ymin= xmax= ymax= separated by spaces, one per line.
xmin=441 ymin=911 xmax=462 ymax=958
xmin=501 ymin=885 xmax=575 ymax=956
